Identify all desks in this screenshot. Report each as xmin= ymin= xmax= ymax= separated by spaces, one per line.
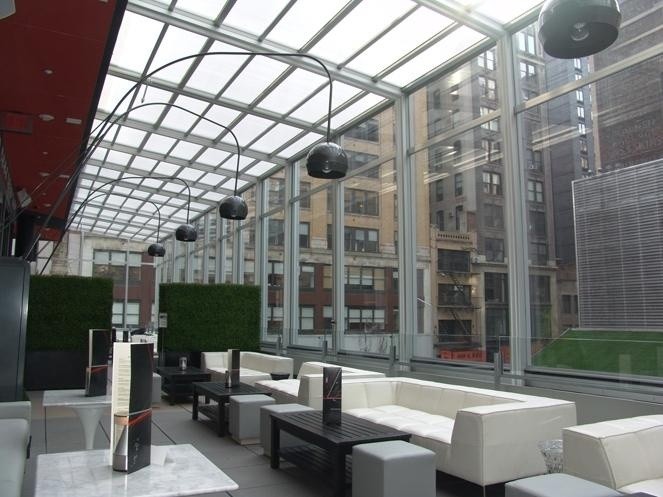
xmin=269 ymin=410 xmax=411 ymax=495
xmin=29 ymin=389 xmax=239 ymax=497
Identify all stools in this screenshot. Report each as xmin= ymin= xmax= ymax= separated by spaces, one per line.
xmin=503 ymin=471 xmax=619 ymax=496
xmin=225 ymin=394 xmax=315 ymax=446
xmin=349 ymin=438 xmax=438 ymax=494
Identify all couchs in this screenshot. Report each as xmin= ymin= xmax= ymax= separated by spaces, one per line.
xmin=202 ymin=349 xmax=295 ymax=392
xmin=315 ymin=374 xmax=577 ymax=496
xmin=252 ymin=358 xmax=385 ymax=413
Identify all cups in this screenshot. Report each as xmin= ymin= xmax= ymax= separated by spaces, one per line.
xmin=178 ymin=356 xmax=187 ymax=371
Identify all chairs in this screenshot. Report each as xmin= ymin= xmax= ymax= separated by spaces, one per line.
xmin=562 ymin=412 xmax=663 ymax=496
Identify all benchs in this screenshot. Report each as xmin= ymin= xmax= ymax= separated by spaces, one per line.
xmin=0 ymin=399 xmax=30 ymax=497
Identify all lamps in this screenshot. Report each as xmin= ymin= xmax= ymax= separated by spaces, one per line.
xmin=535 ymin=0 xmax=621 ymax=59
xmin=22 ymin=47 xmax=348 ymax=273
